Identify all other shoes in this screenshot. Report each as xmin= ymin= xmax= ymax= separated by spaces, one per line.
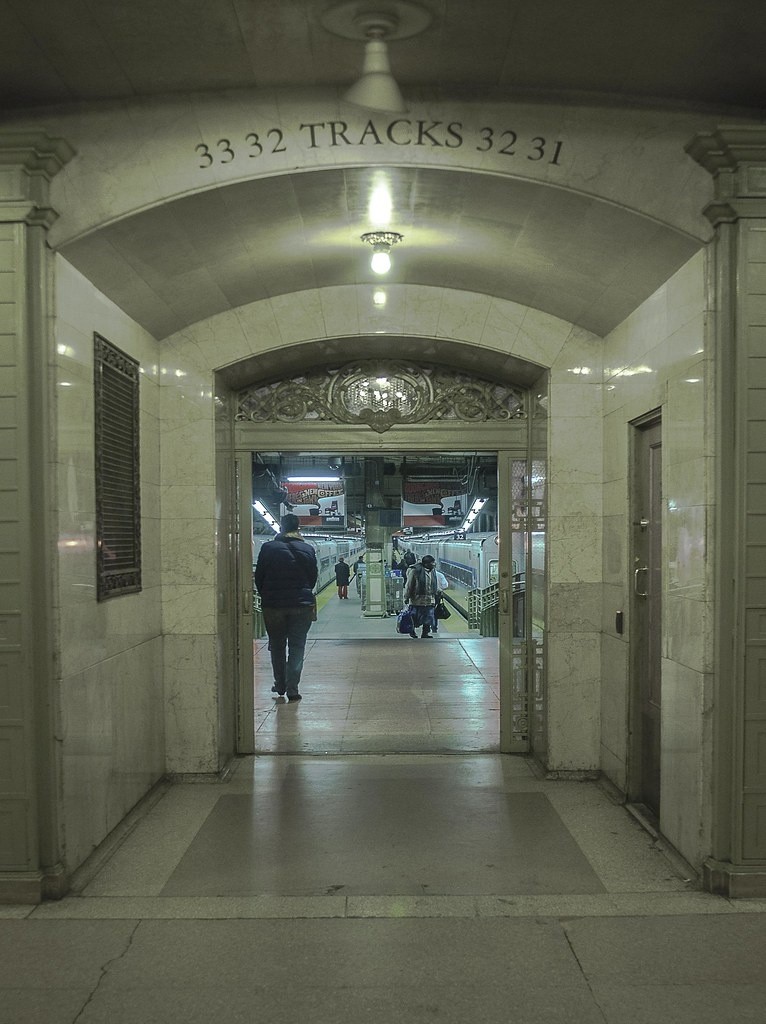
xmin=421 ymin=634 xmax=433 ymax=638
xmin=410 ymin=632 xmax=417 ymax=638
xmin=271 ymin=687 xmax=285 ymax=695
xmin=289 ymin=694 xmax=302 ymax=700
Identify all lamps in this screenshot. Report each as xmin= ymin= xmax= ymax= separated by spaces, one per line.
xmin=340 ymin=12 xmax=409 ymax=115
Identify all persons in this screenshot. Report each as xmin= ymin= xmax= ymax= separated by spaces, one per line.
xmin=254 ymin=514 xmax=318 ymax=701
xmin=354 ymin=556 xmax=365 ymax=589
xmin=393 ymin=549 xmax=448 ymax=638
xmin=335 ymin=557 xmax=350 ymax=600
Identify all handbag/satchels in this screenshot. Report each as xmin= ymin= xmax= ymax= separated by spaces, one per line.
xmin=311 ymin=595 xmax=317 ymax=621
xmin=397 ymin=612 xmax=412 ymax=634
xmin=435 ymin=598 xmax=451 ymax=619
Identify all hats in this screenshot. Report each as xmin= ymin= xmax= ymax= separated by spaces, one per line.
xmin=338 ymin=558 xmax=344 ymax=562
xmin=422 ymin=555 xmax=435 ymax=563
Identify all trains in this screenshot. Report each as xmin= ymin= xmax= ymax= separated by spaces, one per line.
xmin=253 ymin=531 xmax=366 ymax=620
xmin=395 ymin=531 xmax=524 ymax=598
